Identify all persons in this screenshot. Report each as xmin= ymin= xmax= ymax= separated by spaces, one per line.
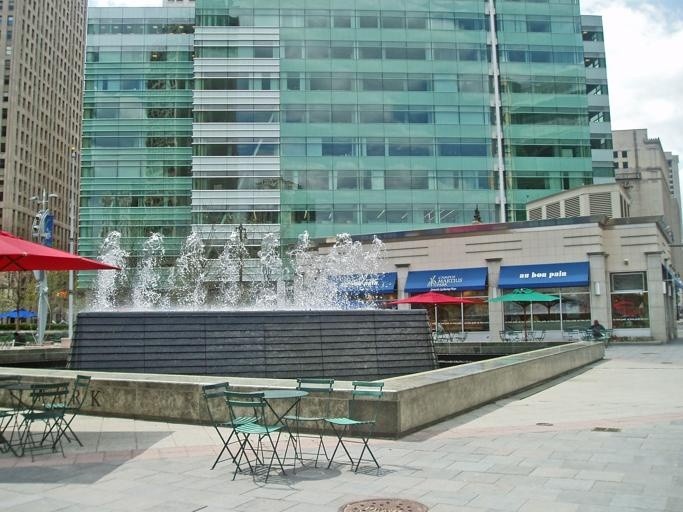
xmin=592 ymin=319 xmax=601 ymax=337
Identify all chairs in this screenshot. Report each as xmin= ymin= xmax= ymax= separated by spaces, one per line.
xmin=201 ymin=378 xmax=383 ymax=483
xmin=568 ymin=328 xmax=613 ymax=349
xmin=433 ymin=330 xmax=467 ymax=343
xmin=0 ymin=374 xmax=91 ymax=462
xmin=498 ymin=329 xmax=545 ymax=341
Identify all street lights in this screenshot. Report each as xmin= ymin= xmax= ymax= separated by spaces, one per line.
xmin=27 ymin=188 xmax=60 ymax=344
xmin=230 ymin=223 xmax=249 ymax=304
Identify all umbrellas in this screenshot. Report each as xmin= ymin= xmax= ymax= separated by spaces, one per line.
xmin=387 ymin=290 xmax=488 ymax=337
xmin=0 ymin=308 xmax=37 ymax=328
xmin=480 ymin=287 xmax=565 ymax=340
xmin=0 ymin=228 xmax=121 ymax=274
